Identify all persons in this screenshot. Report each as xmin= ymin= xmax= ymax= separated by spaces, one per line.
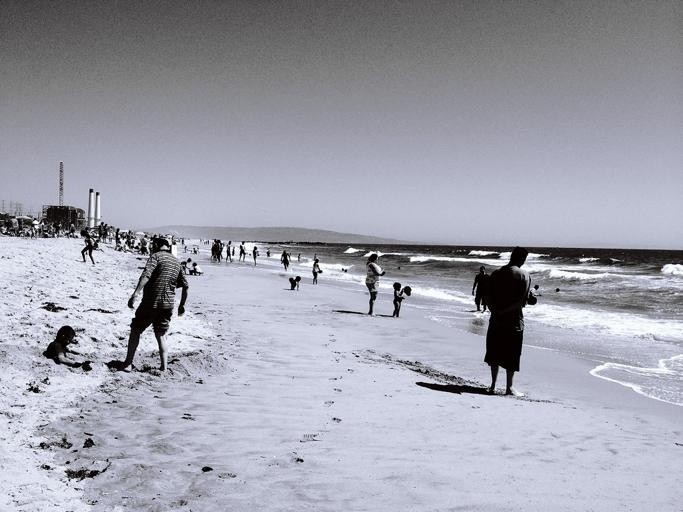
xmin=472 ymin=265 xmax=489 ymax=312
xmin=2 ymin=215 xmax=175 ymax=266
xmin=392 ymin=282 xmax=405 ymax=319
xmin=44 ymin=326 xmax=83 ymax=366
xmin=482 ymin=247 xmax=532 ymax=396
xmin=114 ymin=237 xmax=188 ymax=372
xmin=179 ymin=238 xmax=321 ymax=285
xmin=288 ymin=276 xmax=301 ymax=290
xmin=529 ymin=285 xmax=542 ymax=297
xmin=365 ymin=254 xmax=385 ymax=317
